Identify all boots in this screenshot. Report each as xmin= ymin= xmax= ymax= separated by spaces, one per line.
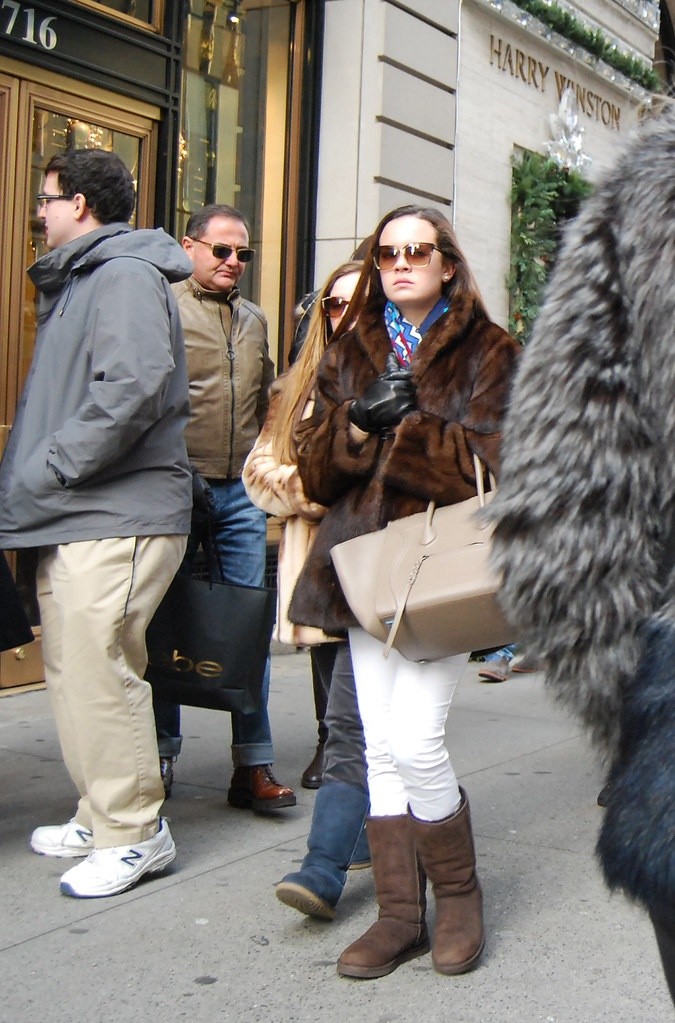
xmin=408 ymin=786 xmax=486 ymax=975
xmin=338 ymin=815 xmax=429 ymax=977
xmin=275 ymin=779 xmax=371 ymax=917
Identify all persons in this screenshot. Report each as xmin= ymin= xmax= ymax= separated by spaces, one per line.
xmin=476 ymin=636 xmax=545 ymax=686
xmin=242 ymin=229 xmax=383 ymax=924
xmin=289 ymin=203 xmax=511 ymax=981
xmin=469 ymin=119 xmax=675 ymax=1012
xmin=0 ymin=150 xmax=195 ymax=899
xmin=143 ymin=205 xmax=298 ymax=813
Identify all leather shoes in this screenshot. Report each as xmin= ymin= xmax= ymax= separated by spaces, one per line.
xmin=302 ymin=724 xmax=328 ymax=788
xmin=228 ymin=765 xmax=297 ymax=811
xmin=161 ymin=757 xmax=174 ymax=799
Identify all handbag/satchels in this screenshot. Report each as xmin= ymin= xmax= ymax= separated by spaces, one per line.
xmin=329 ymin=452 xmax=522 ymax=665
xmin=143 ymin=521 xmax=278 ymax=715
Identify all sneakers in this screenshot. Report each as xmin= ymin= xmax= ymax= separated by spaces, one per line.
xmin=478 ymin=655 xmax=510 ymax=681
xmin=60 ymin=816 xmax=177 ymax=897
xmin=30 ymin=818 xmax=96 ymax=859
xmin=512 ymin=655 xmax=548 ymax=673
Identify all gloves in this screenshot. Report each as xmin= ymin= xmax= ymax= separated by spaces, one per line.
xmin=347 ymin=353 xmax=417 ymax=439
xmin=190 ymin=465 xmax=216 ymax=515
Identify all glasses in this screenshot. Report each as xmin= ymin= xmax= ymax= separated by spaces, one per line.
xmin=322 ymin=295 xmax=350 ymax=319
xmin=35 ymin=193 xmax=75 ymax=208
xmin=372 ymin=243 xmax=446 ymax=270
xmin=190 ymin=237 xmax=256 ymax=262
